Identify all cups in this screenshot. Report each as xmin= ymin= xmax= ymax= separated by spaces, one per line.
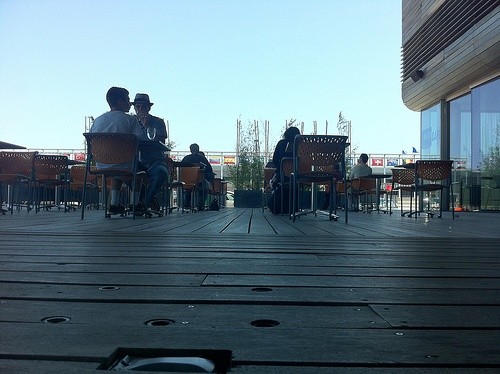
xmin=147 ymin=128 xmax=156 ymax=141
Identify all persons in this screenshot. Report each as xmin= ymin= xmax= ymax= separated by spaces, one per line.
xmin=88 ymin=87 xmax=147 ymax=210
xmin=129 ymin=93 xmax=168 ymax=214
xmin=348 ymin=154 xmax=372 ymax=210
xmin=269 ymin=127 xmax=303 ymax=214
xmin=179 ymin=144 xmax=214 ymax=212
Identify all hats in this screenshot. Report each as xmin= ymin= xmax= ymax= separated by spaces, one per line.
xmin=129 ymin=93 xmax=154 ymax=106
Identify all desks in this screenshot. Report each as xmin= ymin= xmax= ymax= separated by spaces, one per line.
xmin=119 ymin=141 xmax=173 ymax=218
xmin=173 ymin=161 xmax=194 ymax=214
xmin=361 ymin=173 xmax=392 ymax=214
xmin=39 ymin=158 xmax=85 ymax=211
xmin=394 ymin=163 xmax=453 ymax=218
xmin=283 ymin=140 xmax=353 ymax=221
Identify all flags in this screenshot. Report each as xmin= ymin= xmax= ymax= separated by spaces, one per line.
xmin=413 ymin=147 xmax=418 ymax=153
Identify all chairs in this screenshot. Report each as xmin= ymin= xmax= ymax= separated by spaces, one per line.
xmin=212 ymin=178 xmax=221 ymax=208
xmin=407 ymin=159 xmax=457 ymax=222
xmin=94 ymin=172 xmax=114 ymax=208
xmin=65 ymin=163 xmax=98 ymax=211
xmin=220 ymin=184 xmax=228 ymax=209
xmin=179 ymin=164 xmax=200 ymax=212
xmin=351 ymin=178 xmax=360 ymax=212
xmin=203 ymin=173 xmax=214 ymax=209
xmin=28 ymin=153 xmax=69 ymax=212
xmin=336 ymin=180 xmax=346 ymax=211
xmin=78 ymin=130 xmax=145 ymax=222
xmin=390 ymin=169 xmax=421 ymax=216
xmin=358 ymin=177 xmax=389 ymax=214
xmin=0 ymin=150 xmax=40 ymax=215
xmin=290 ymin=130 xmax=350 ymax=222
xmin=272 ymin=155 xmax=318 ymax=217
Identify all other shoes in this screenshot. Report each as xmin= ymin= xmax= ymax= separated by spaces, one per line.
xmin=109 ymin=204 xmax=124 ymax=215
